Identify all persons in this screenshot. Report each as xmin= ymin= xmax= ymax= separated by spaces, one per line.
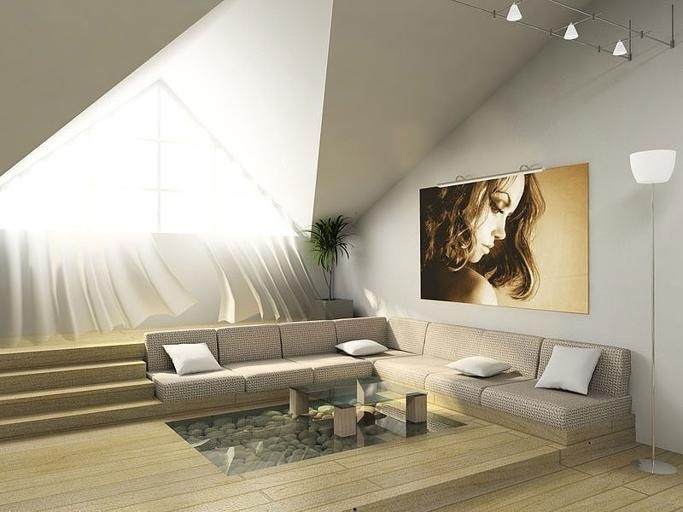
xmin=421 ymin=173 xmax=547 ymax=306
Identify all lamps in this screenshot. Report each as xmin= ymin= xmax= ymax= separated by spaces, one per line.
xmin=629 ymin=150 xmax=676 ymax=476
xmin=506 ymin=0 xmax=522 ymax=21
xmin=610 ymin=38 xmax=627 ymax=55
xmin=435 ymin=165 xmax=544 ymax=189
xmin=563 ymin=21 xmax=578 ymax=41
xmin=161 ymin=342 xmax=222 ymax=376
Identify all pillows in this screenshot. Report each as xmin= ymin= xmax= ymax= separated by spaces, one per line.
xmin=334 ymin=339 xmax=388 ymax=357
xmin=534 ymin=345 xmax=601 ymax=396
xmin=444 ymin=357 xmax=511 ymax=378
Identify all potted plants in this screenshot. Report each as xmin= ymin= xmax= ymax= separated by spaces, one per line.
xmin=297 ymin=215 xmax=358 ymax=319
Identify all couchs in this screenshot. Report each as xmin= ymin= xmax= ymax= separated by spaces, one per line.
xmin=144 ymin=317 xmax=632 ymax=447
